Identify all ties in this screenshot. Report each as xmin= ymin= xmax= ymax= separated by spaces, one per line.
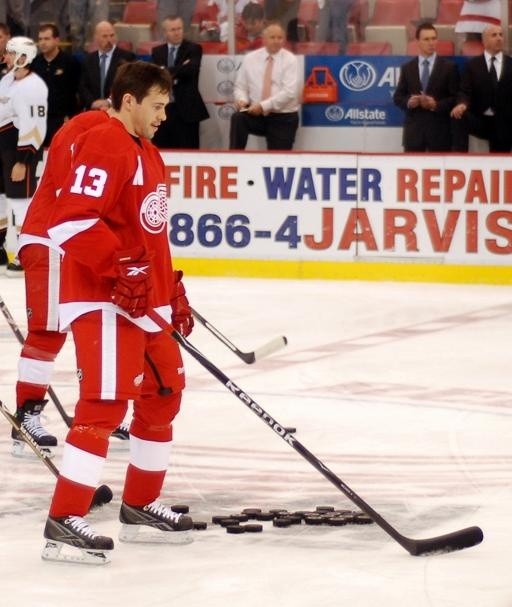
xmin=490 ymin=56 xmax=498 ymax=86
xmin=100 ymin=53 xmax=108 ymax=99
xmin=261 ymin=55 xmax=273 ymax=101
xmin=421 ymin=59 xmax=431 ymax=92
xmin=168 ymin=47 xmax=175 ymax=67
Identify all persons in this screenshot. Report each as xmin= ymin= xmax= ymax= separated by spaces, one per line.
xmin=1 ymin=0 xmax=352 ymax=152
xmin=41 ymin=61 xmax=198 ymax=567
xmin=0 ymin=34 xmax=49 ymax=278
xmin=451 ymin=26 xmax=511 ymax=153
xmin=10 ymin=105 xmax=131 ymax=461
xmin=394 ymin=23 xmax=457 ymax=153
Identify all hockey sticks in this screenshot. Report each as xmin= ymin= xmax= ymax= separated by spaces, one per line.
xmin=143 ymin=304 xmax=484 ymax=557
xmin=191 ymin=308 xmax=287 ymax=364
xmin=0 ymin=400 xmax=113 ymax=507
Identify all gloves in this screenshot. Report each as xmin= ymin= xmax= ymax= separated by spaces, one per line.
xmin=170 ymin=270 xmax=194 ymax=338
xmin=110 ymin=246 xmax=152 ymax=318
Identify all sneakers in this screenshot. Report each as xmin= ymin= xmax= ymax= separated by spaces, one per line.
xmin=111 ymin=426 xmax=131 ymax=439
xmin=8 ymin=255 xmax=24 ymax=271
xmin=120 ymin=500 xmax=193 ymax=532
xmin=43 ymin=515 xmax=113 ymax=549
xmin=12 ymin=400 xmax=58 ymax=446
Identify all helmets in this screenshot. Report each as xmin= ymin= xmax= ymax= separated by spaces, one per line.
xmin=6 ymin=36 xmax=37 ymax=68
